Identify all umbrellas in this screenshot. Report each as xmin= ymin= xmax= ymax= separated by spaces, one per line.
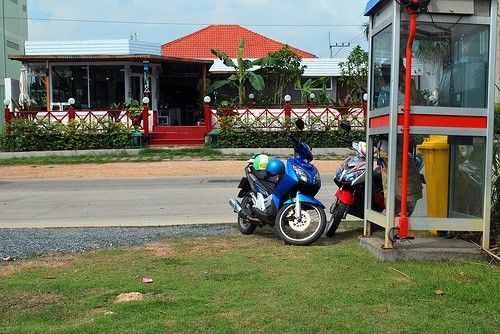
xmin=19 ymin=65 xmax=30 ymax=107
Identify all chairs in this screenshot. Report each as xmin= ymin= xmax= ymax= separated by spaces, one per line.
xmin=158 ymin=108 xmax=169 ymax=126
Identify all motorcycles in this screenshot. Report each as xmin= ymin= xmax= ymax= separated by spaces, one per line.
xmin=325 ymin=122 xmax=426 ymax=237
xmin=228 ymin=118 xmax=326 ymax=247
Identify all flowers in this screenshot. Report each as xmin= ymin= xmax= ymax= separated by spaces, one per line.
xmin=193 ymin=110 xmax=204 ymax=122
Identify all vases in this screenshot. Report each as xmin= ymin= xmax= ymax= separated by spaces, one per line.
xmin=196 ymin=120 xmax=201 ymax=125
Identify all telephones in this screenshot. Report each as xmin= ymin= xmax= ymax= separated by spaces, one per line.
xmin=399 ymin=73 xmax=411 ymax=83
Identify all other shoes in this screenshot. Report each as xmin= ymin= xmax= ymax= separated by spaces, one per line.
xmin=394 ymin=234 xmax=414 ymax=239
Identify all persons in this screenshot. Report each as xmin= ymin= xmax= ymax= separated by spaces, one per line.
xmin=377 ymin=56 xmax=424 ymax=240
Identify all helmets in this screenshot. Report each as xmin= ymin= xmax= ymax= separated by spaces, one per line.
xmin=265 ymin=159 xmax=285 ymax=176
xmin=253 ymin=154 xmax=271 ymax=170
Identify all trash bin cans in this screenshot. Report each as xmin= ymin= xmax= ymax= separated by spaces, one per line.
xmin=417 ymin=134 xmax=450 ymax=235
